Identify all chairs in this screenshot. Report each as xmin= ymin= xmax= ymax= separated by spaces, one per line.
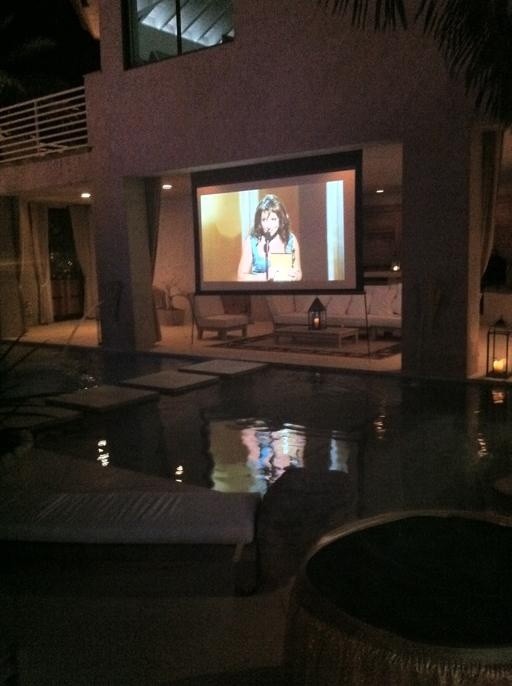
xmin=187 ymin=291 xmax=249 ymax=340
xmin=1 ymin=486 xmax=263 ymax=597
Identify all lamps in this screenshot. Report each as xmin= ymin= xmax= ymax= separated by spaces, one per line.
xmin=486 ymin=315 xmax=512 ymax=379
xmin=308 ymin=295 xmax=328 ymax=331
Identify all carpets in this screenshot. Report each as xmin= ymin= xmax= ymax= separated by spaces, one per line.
xmin=203 ymin=333 xmax=401 ymax=361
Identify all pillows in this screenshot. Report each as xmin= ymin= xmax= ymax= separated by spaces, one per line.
xmin=271 ymin=282 xmax=403 ymax=316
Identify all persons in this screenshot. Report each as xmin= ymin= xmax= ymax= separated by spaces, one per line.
xmin=234 ymin=192 xmax=304 ymax=282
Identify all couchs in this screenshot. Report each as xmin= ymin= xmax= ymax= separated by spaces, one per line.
xmin=267 ymin=301 xmax=403 ymax=340
